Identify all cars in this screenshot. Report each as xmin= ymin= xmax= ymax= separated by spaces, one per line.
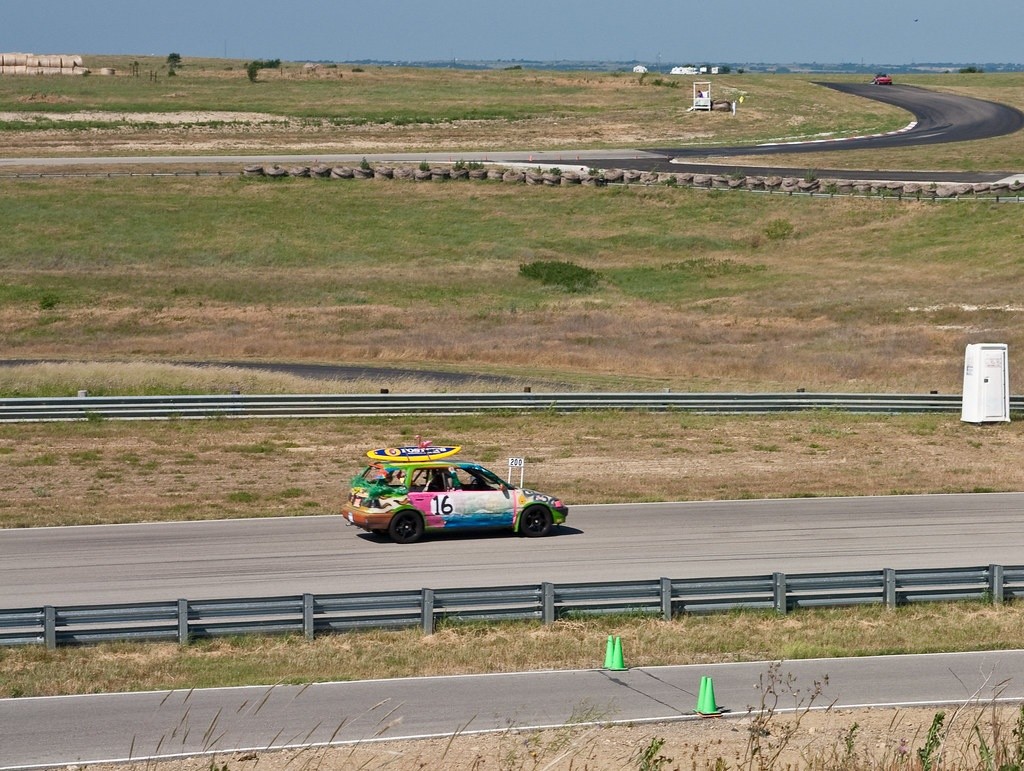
xmin=874 ymin=75 xmax=892 ymax=85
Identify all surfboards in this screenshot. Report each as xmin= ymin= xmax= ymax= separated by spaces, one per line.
xmin=365 ymin=446 xmax=462 ymax=462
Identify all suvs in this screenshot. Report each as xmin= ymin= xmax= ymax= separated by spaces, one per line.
xmin=341 ymin=461 xmax=569 ymax=543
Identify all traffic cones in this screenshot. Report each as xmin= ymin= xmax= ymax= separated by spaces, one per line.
xmin=699 ymin=677 xmax=723 ymax=717
xmin=609 ymin=637 xmax=629 ymax=670
xmin=692 ymin=677 xmax=707 ymax=712
xmin=601 ymin=635 xmax=614 ymax=669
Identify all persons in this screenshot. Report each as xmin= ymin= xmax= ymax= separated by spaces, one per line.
xmin=698 ymin=90 xmax=703 ymax=98
